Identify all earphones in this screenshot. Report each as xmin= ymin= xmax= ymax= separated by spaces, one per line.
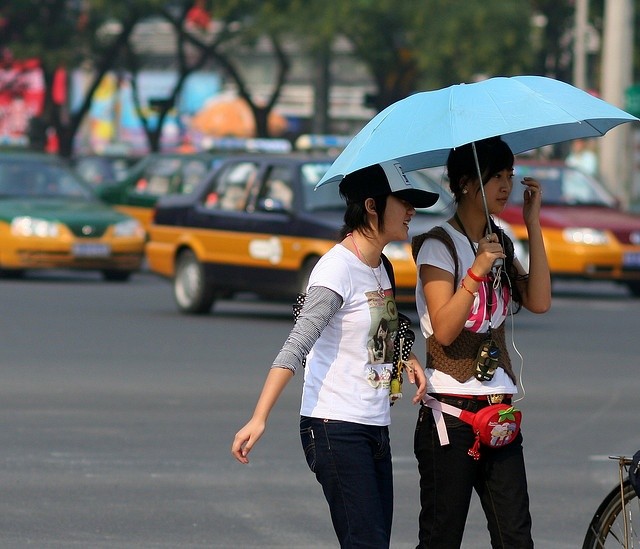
xmin=462 ymin=189 xmax=468 ymax=194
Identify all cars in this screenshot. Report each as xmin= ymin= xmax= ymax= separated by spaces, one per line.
xmin=95 ymin=137 xmax=292 ymax=231
xmin=147 ymin=135 xmax=524 ymax=313
xmin=490 ymin=160 xmax=640 ymax=296
xmin=0 ymin=135 xmax=145 ymax=280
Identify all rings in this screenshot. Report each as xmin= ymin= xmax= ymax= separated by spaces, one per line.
xmin=539 ymin=189 xmax=543 ymax=194
xmin=486 ymin=234 xmax=493 ymax=241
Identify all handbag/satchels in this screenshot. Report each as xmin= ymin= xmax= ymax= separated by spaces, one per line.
xmin=292 ymin=253 xmax=415 ymax=406
xmin=421 ymin=393 xmax=522 ymax=460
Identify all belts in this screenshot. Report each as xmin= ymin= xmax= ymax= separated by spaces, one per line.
xmin=440 ymin=393 xmax=513 ymax=403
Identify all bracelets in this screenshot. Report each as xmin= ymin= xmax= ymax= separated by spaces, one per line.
xmin=466 ymin=268 xmax=485 ymax=282
xmin=461 ymin=278 xmax=479 ymax=297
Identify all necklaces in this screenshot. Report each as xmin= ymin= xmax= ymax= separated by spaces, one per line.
xmin=351 ymin=234 xmax=386 ymax=299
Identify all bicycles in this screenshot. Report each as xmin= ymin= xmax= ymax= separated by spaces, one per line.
xmin=579 ymin=448 xmax=639 ymax=542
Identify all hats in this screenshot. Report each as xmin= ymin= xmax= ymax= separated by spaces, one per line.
xmin=339 ymin=159 xmax=439 ymax=208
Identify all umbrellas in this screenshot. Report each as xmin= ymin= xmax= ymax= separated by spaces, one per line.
xmin=313 ymin=76 xmax=640 ymax=266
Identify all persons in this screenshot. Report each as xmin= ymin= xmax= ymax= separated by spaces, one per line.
xmin=414 ymin=138 xmax=553 ymax=548
xmin=230 ymin=159 xmax=429 ymax=549
